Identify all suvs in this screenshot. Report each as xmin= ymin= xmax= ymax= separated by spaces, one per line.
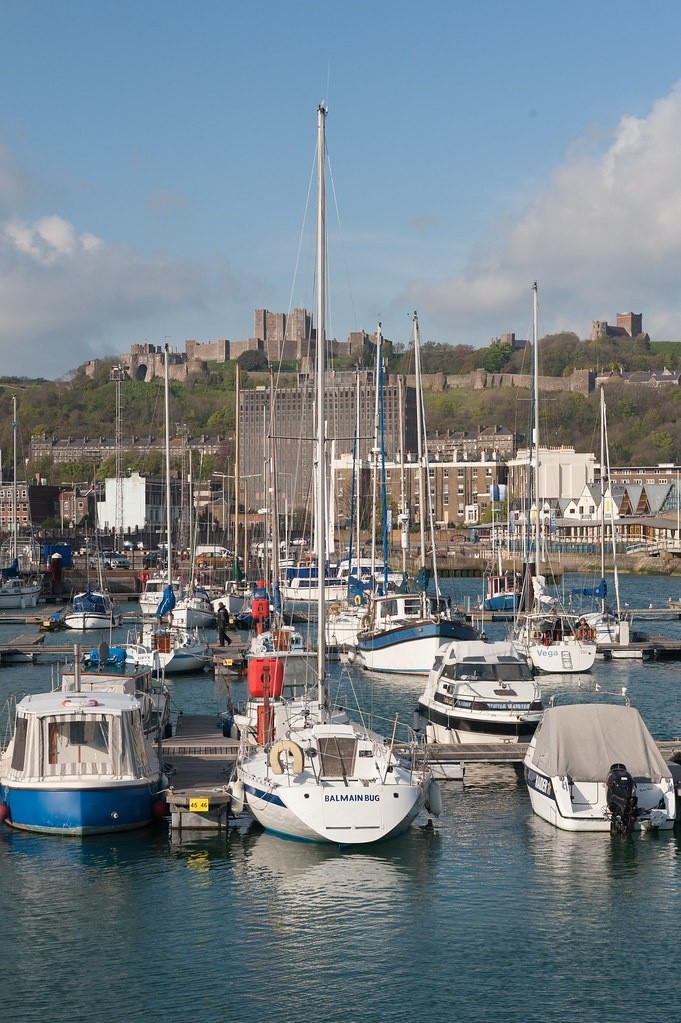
xmin=143 ymin=551 xmax=179 ymax=570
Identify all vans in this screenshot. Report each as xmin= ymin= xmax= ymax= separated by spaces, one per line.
xmin=89 ymin=551 xmax=130 ymax=570
xmin=195 ymin=545 xmax=243 ymax=564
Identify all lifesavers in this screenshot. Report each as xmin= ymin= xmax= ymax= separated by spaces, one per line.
xmin=576 ymin=626 xmax=591 ymax=641
xmin=328 ymin=604 xmax=341 ymax=614
xmin=354 ymin=595 xmax=361 ymax=605
xmin=268 ymin=739 xmax=305 ymax=775
xmin=61 ymin=699 xmax=98 ymax=707
xmin=362 ymin=616 xmax=370 ymax=627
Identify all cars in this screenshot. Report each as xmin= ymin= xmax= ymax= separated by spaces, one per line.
xmin=133 ymin=541 xmax=149 ymax=550
xmin=365 ymin=537 xmax=388 ymax=546
xmin=153 ymin=540 xmax=174 ymax=550
xmin=195 ymin=551 xmax=239 ymax=568
xmin=122 ymin=540 xmax=133 ymax=550
xmin=289 ymin=537 xmax=308 ymax=546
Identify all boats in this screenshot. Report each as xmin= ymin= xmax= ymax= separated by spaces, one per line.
xmin=51 ymin=603 xmax=174 ymax=741
xmin=413 ymin=571 xmax=543 ymax=744
xmin=0 ymin=644 xmax=168 ymax=835
xmin=522 ymin=677 xmax=676 ymax=840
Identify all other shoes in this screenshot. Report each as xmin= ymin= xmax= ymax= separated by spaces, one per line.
xmin=227 ymin=639 xmax=232 ymax=645
xmin=218 ymin=644 xmax=225 ymax=647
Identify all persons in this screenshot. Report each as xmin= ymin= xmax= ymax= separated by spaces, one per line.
xmin=216 ymin=603 xmax=233 ymax=647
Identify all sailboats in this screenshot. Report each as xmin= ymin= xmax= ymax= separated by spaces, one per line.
xmin=229 ymin=99 xmax=443 ymax=846
xmin=0 ymin=393 xmax=47 ymax=607
xmin=121 ymin=281 xmax=650 ymax=675
xmin=59 ymin=461 xmax=122 ymax=629
